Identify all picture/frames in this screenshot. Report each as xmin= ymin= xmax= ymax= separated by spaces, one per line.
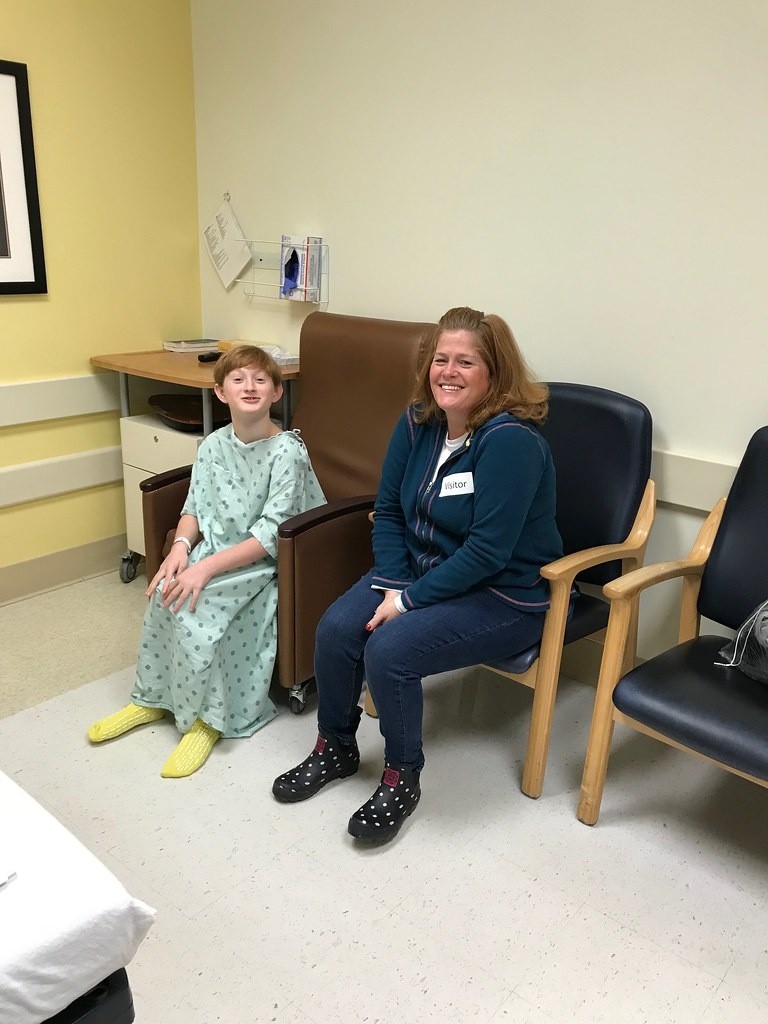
xmin=0 ymin=59 xmax=47 ymax=295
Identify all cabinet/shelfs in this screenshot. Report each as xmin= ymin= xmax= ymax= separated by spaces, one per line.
xmin=118 ymin=411 xmax=282 ymax=557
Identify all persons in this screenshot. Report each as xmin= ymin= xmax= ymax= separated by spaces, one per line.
xmin=86 ymin=345 xmax=328 ymax=779
xmin=270 ymin=305 xmax=564 ymax=846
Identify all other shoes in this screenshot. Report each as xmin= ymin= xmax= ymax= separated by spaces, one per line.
xmin=273 ymin=728 xmax=360 ymax=801
xmin=348 ymin=760 xmax=422 ymax=843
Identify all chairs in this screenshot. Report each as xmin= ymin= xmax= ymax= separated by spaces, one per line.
xmin=137 ymin=311 xmax=438 ymax=715
xmin=575 ymin=424 xmax=767 ymax=827
xmin=364 ymin=382 xmax=654 ymax=799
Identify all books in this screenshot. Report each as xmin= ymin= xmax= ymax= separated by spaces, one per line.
xmin=163 ymin=339 xmax=220 ymax=352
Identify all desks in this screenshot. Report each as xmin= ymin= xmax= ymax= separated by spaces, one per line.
xmin=88 ymin=350 xmax=299 ymax=583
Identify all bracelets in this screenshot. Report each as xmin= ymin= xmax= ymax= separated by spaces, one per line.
xmin=173 ymin=536 xmax=191 ymax=554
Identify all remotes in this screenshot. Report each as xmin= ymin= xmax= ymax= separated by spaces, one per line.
xmin=198 ymin=352 xmax=224 ymax=362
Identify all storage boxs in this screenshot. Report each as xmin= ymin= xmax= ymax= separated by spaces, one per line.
xmin=279 ymin=233 xmax=323 ymax=303
